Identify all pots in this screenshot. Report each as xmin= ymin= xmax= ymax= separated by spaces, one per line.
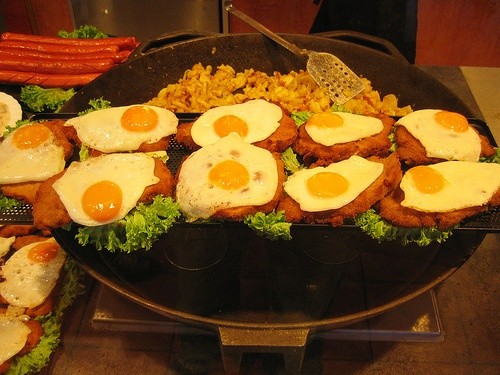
xmin=47 ymin=30 xmax=498 ymax=374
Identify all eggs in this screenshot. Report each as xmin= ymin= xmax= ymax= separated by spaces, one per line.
xmin=0 ymin=314 xmax=32 ymax=365
xmin=0 ymin=90 xmax=500 ymax=226
xmin=0 ymin=236 xmax=67 ymax=309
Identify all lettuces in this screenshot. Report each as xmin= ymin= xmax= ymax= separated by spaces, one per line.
xmin=244 ymin=209 xmax=294 ymax=240
xmin=355 ymin=208 xmax=454 ymax=247
xmin=74 ymin=194 xmax=182 ymax=254
xmin=20 ymin=84 xmax=77 ymax=114
xmin=57 ymin=25 xmax=108 ymax=38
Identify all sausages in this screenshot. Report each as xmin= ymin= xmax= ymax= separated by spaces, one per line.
xmin=0 ymin=31 xmax=141 ymax=87
